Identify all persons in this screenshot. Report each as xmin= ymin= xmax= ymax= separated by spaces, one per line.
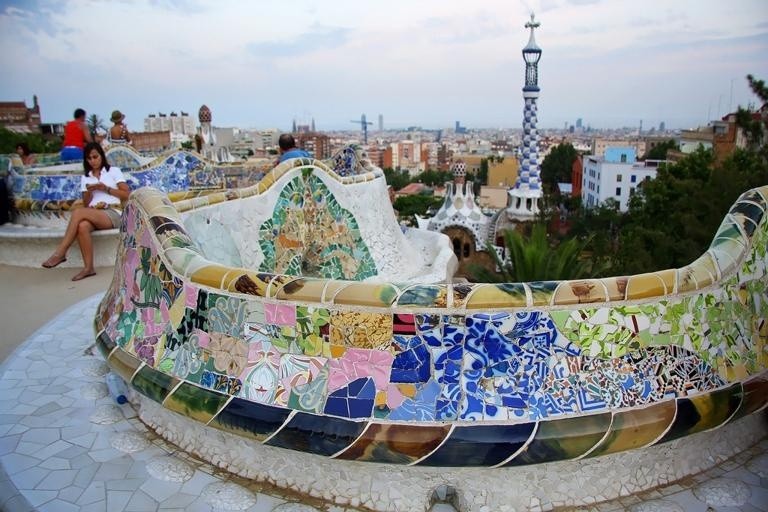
xmin=41 ymin=141 xmax=131 ymax=281
xmin=106 ymin=111 xmax=131 ymax=146
xmin=272 ymin=132 xmax=312 ymax=165
xmin=60 ymin=108 xmax=93 ymax=164
xmin=14 ymin=142 xmax=39 ymax=165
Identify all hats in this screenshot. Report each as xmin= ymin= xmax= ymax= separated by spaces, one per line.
xmin=109 ymin=110 xmax=125 ymax=124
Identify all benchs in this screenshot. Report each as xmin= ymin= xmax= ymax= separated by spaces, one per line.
xmin=2 ymin=174 xmax=122 ymax=274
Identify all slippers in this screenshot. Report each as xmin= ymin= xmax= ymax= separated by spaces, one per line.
xmin=71 ymin=268 xmax=97 ymax=282
xmin=41 ymin=251 xmax=66 ymax=269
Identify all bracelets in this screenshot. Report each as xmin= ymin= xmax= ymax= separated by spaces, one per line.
xmin=104 ymin=186 xmax=110 ymax=194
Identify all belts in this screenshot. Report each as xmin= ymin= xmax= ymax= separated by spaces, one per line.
xmin=63 ymin=145 xmax=78 ymax=149
